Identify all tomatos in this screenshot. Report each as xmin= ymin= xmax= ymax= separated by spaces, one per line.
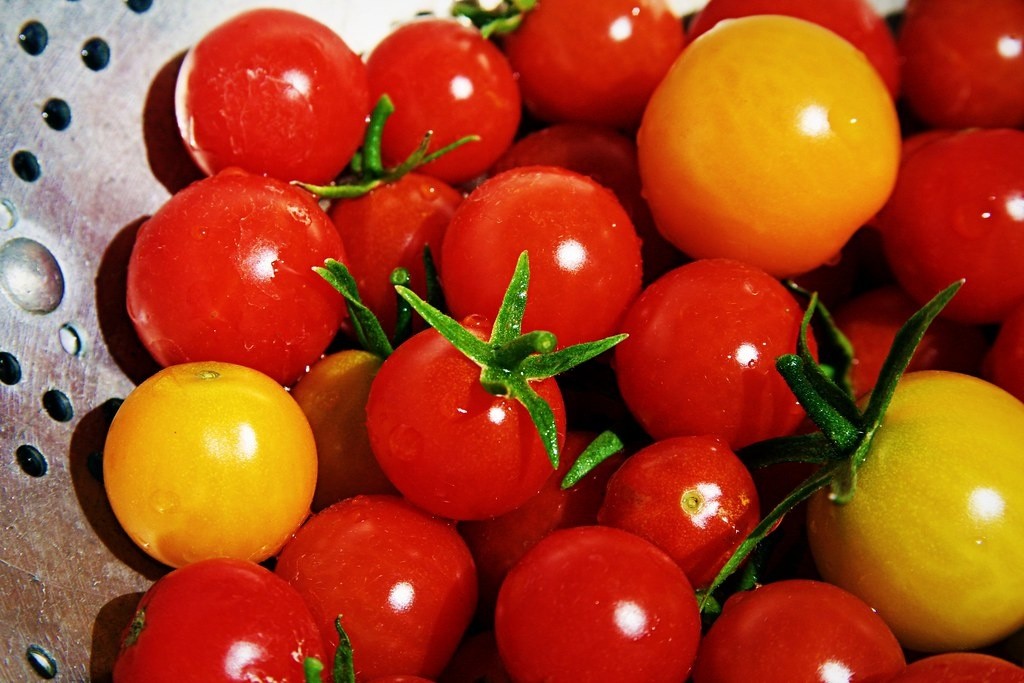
xmin=102 ymin=0 xmax=1024 ymax=683
